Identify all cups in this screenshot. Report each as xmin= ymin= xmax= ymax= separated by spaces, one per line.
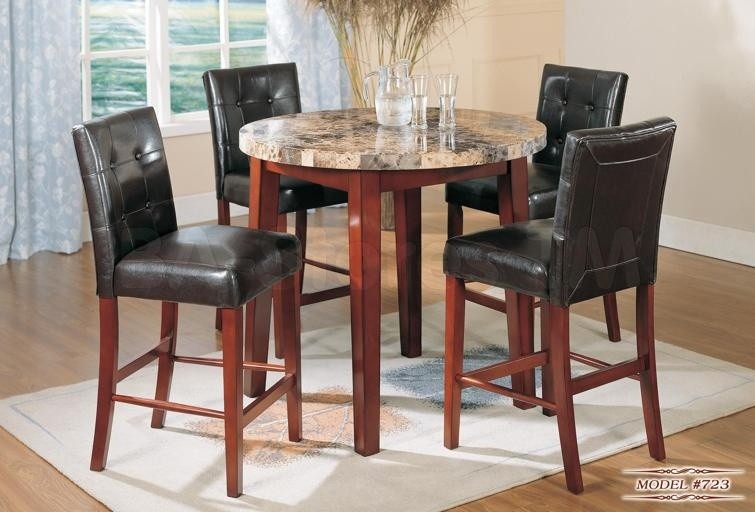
xmin=376 ymin=126 xmax=414 ymax=155
xmin=436 ymin=74 xmax=458 ymax=127
xmin=412 ymin=75 xmax=430 ymax=126
xmin=363 ymin=60 xmax=413 ymax=126
xmin=439 ymin=127 xmax=456 ymax=153
xmin=412 ymin=126 xmax=428 ymax=153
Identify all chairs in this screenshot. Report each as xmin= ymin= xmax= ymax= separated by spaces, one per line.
xmin=201 ymin=61 xmax=422 ymax=359
xmin=445 ymin=62 xmax=630 ymax=342
xmin=71 ymin=105 xmax=304 ymax=497
xmin=443 ymin=115 xmax=678 ymax=493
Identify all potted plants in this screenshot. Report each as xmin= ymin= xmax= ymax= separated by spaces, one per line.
xmin=306 ymin=1 xmax=466 ymax=229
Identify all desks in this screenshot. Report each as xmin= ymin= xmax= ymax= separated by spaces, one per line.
xmin=238 ymin=107 xmax=548 ymax=456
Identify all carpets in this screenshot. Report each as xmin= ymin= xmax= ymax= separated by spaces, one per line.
xmin=0 ymin=285 xmax=755 ymax=512
